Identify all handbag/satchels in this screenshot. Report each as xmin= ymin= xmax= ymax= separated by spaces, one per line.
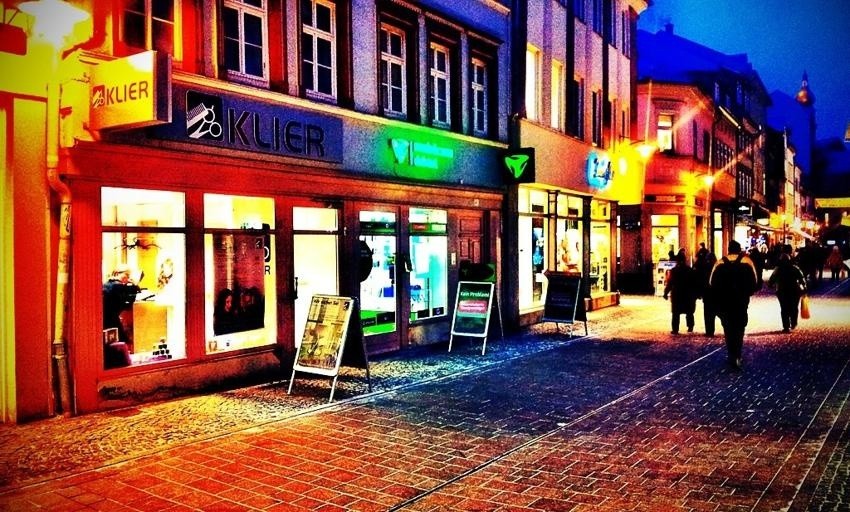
xmin=800 ymin=293 xmax=810 ymax=319
xmin=796 ymin=280 xmax=805 ymax=294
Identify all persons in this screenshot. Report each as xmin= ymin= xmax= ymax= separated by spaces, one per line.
xmin=707 ymin=239 xmax=759 ymax=371
xmin=238 ymin=285 xmax=265 ymax=333
xmin=696 ymin=251 xmax=727 ymax=337
xmin=744 ymin=237 xmax=841 ymax=290
xmin=767 ymin=253 xmax=807 ymax=332
xmin=662 ymin=251 xmax=699 ymax=334
xmin=212 ymin=287 xmax=240 ymax=337
xmin=103 ymin=263 xmax=141 ymax=345
xmin=668 ymin=247 xmax=686 ymax=262
xmin=695 ymin=242 xmax=709 ymax=260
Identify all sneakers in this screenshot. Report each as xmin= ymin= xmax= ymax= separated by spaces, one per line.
xmin=672 ymin=325 xmax=714 ymax=336
xmin=727 ymin=356 xmax=744 ymax=370
xmin=782 ymin=323 xmax=798 ymax=333
